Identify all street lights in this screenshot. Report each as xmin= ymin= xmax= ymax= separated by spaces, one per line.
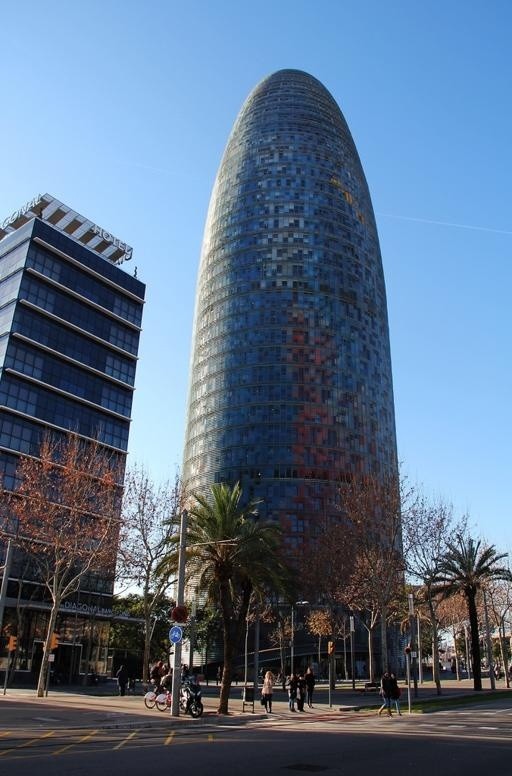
xmin=290 ymin=600 xmax=309 ymax=676
xmin=171 ymin=509 xmax=240 ymax=717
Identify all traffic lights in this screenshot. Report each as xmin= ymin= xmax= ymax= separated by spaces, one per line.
xmin=327 ymin=641 xmax=336 ymax=656
xmin=8 ymin=636 xmax=17 ymax=653
xmin=50 ymin=633 xmax=60 ymax=649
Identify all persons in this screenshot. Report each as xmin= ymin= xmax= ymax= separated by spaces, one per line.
xmin=304 ymin=667 xmax=315 ymax=709
xmin=296 ymin=674 xmax=306 ymax=713
xmin=261 ymin=671 xmax=275 ymax=714
xmin=286 ymin=675 xmax=298 ymax=713
xmin=160 ymin=668 xmax=173 ymax=694
xmin=150 ymin=661 xmax=165 ymax=695
xmin=116 ymin=665 xmax=129 ymax=697
xmin=388 ymin=672 xmax=403 ymax=717
xmin=377 ymin=672 xmax=394 ymax=719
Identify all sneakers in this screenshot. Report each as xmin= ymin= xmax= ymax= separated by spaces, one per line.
xmin=290 ymin=704 xmax=314 ymax=712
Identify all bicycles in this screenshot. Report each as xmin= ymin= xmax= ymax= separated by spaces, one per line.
xmin=144 ymin=686 xmax=171 ymax=712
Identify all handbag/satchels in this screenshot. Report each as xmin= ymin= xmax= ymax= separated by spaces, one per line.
xmin=261 ymin=698 xmax=266 ymax=705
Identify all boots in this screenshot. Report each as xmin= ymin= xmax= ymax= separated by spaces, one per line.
xmin=378 ymin=707 xmax=392 ymax=716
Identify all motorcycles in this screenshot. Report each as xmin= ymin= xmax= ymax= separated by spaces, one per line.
xmin=179 ymin=681 xmax=204 ymax=719
